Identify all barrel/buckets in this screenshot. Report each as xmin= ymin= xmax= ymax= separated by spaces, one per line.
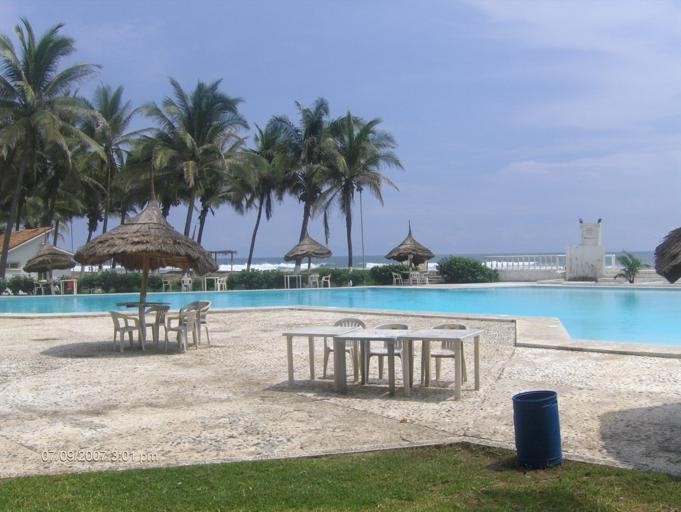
xmin=513 ymin=389 xmax=562 ymax=469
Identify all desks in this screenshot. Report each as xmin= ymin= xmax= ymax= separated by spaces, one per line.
xmin=401 ymin=271 xmax=420 ymax=273
xmin=398 ymin=328 xmax=483 ymax=400
xmin=116 ymin=298 xmax=172 ymax=307
xmin=283 ymin=275 xmax=302 ymax=288
xmin=334 ymin=328 xmax=411 ymax=395
xmin=202 ymin=276 xmax=221 ymax=290
xmin=280 ymin=324 xmax=359 ymax=386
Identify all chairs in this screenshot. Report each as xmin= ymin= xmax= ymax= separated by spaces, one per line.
xmin=216 ymin=276 xmax=228 ymax=290
xmin=165 ymin=310 xmax=196 ymax=352
xmin=310 ymin=274 xmax=319 ymax=288
xmin=322 ymin=272 xmax=331 ymax=287
xmin=110 ymin=311 xmax=142 ymax=352
xmin=420 ymin=323 xmax=466 ymax=385
xmin=409 ymin=272 xmax=419 ymax=285
xmin=390 ymin=272 xmax=402 ymax=285
xmin=361 ymin=323 xmax=409 ymax=385
xmin=144 ymin=305 xmax=171 ymax=347
xmin=321 ymin=318 xmax=366 ymax=379
xmin=177 ymin=300 xmax=211 ymax=347
xmin=161 ymin=275 xmax=172 ymax=291
xmin=33 ymin=279 xmax=60 ymax=296
